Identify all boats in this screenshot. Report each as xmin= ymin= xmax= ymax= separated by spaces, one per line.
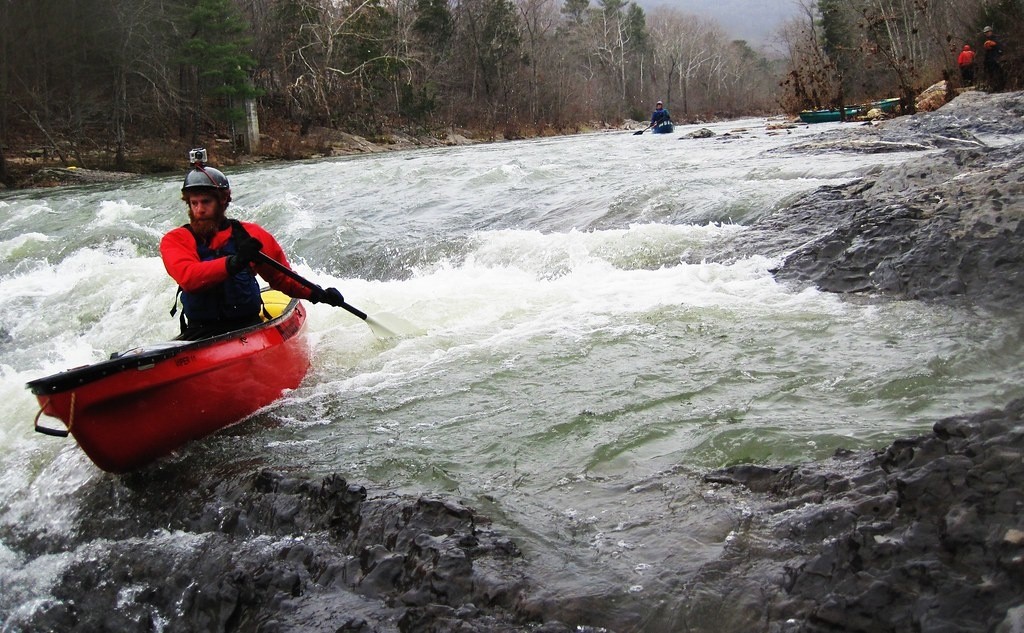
xmin=651 ymin=117 xmax=673 ymax=134
xmin=796 ymin=97 xmax=903 ymax=124
xmin=22 ymin=283 xmax=313 ymax=474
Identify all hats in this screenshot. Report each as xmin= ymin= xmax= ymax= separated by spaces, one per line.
xmin=983 ymin=26 xmax=993 ymax=33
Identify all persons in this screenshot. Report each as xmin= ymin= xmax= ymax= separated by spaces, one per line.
xmin=161 ymin=166 xmax=343 ymax=343
xmin=958 ymin=45 xmax=977 ymax=88
xmin=983 ymin=26 xmax=1005 ymax=92
xmin=649 ymin=101 xmax=670 ymax=127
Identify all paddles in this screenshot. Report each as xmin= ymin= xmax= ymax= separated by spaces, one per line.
xmin=255 ymin=250 xmax=423 ymax=340
xmin=633 ymin=117 xmax=665 ymax=135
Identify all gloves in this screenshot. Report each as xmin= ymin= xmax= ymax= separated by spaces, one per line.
xmin=226 ymin=237 xmax=265 ymax=276
xmin=309 ymin=285 xmax=344 ymax=307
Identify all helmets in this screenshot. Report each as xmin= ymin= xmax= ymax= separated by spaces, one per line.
xmin=657 ymin=101 xmax=663 ymax=105
xmin=182 ymin=165 xmax=230 ymax=191
xmin=963 ymin=45 xmax=970 ymax=50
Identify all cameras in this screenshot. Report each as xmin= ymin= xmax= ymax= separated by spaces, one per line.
xmin=190 ymin=148 xmax=208 ymax=163
